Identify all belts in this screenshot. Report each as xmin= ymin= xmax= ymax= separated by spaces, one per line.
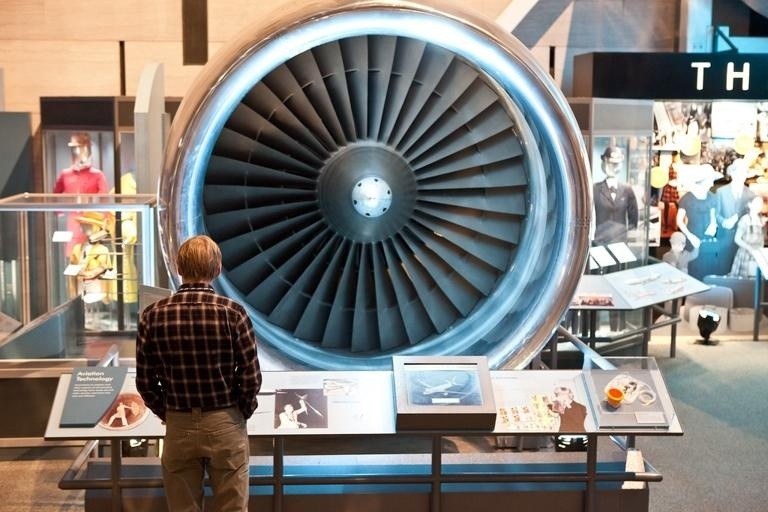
xmin=164 ymin=403 xmax=240 ymax=415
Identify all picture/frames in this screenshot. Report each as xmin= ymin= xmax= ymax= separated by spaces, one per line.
xmin=391 ymin=356 xmax=497 ymax=431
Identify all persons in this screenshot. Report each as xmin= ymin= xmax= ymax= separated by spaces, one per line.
xmin=540 ymin=387 xmax=586 ymax=431
xmin=136 ymin=236 xmax=262 ymax=512
xmin=52 ymin=133 xmax=137 ymax=328
xmin=593 ymin=147 xmax=764 ymax=314
xmin=278 ymin=394 xmax=307 ymax=428
xmin=105 ymin=402 xmax=132 ymax=427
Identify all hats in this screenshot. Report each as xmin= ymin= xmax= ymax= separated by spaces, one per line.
xmin=66 ymin=134 xmax=94 ymax=149
xmin=75 ymin=211 xmax=108 ymax=228
xmin=677 ymin=131 xmax=765 ymax=182
xmin=599 ymin=145 xmax=625 ymax=165
